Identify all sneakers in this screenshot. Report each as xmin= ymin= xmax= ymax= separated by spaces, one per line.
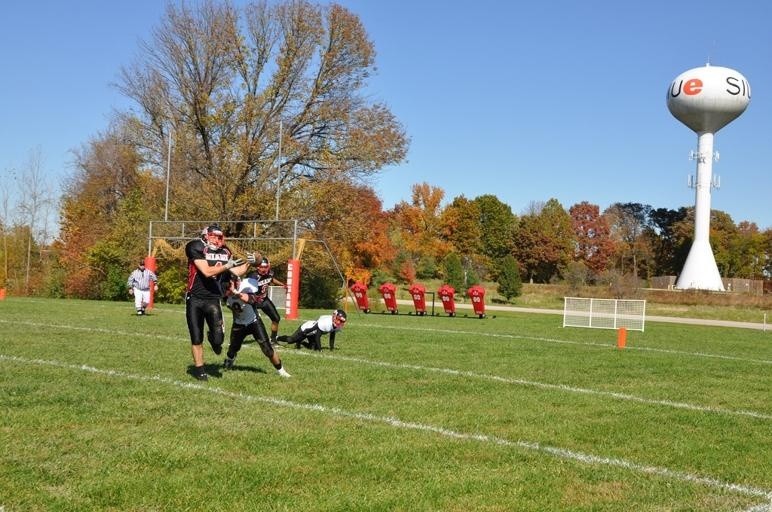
xmin=207 ymin=332 xmax=221 ymax=355
xmin=276 ymin=367 xmax=290 ymax=378
xmin=138 ymin=310 xmax=142 ymax=314
xmin=195 ymin=368 xmax=207 ymax=382
xmin=141 ymin=307 xmax=145 ymax=312
xmin=224 ymin=352 xmax=236 ymax=369
xmin=270 ymin=335 xmax=278 ymax=344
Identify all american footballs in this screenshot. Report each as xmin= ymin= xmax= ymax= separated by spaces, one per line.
xmin=251 ymin=251 xmax=262 ymax=267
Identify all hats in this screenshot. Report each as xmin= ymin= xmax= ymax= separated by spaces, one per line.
xmin=140 ymin=260 xmax=144 ymax=266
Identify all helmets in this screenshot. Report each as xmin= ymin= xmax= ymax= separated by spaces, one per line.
xmin=257 ymin=257 xmax=270 ymax=275
xmin=200 ymin=226 xmax=224 ymax=251
xmin=332 ymin=309 xmax=347 ymax=327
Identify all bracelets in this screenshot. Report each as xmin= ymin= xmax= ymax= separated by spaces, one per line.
xmin=153 ymin=283 xmax=157 ymax=285
xmin=129 ymin=287 xmax=132 ymax=289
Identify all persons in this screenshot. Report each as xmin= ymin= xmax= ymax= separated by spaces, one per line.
xmin=185 ymin=226 xmax=293 ymax=381
xmin=269 ymin=308 xmax=347 ymax=353
xmin=127 ymin=259 xmax=159 ymax=315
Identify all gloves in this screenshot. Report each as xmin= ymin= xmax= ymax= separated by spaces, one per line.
xmin=226 ymin=255 xmax=245 ymax=269
xmin=248 ymin=254 xmax=255 ymax=263
